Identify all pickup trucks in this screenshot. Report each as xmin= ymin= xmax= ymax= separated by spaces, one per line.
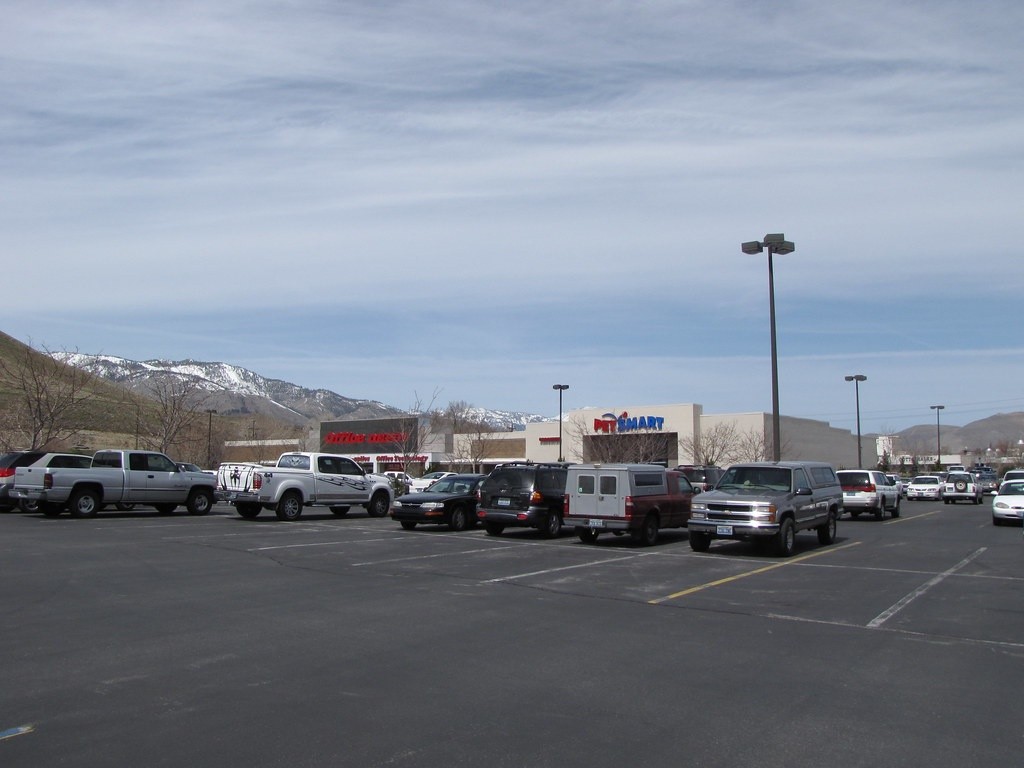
xmin=8 ymin=447 xmax=219 ymax=519
xmin=562 ymin=461 xmax=700 ymax=548
xmin=215 ymin=451 xmax=395 ymax=522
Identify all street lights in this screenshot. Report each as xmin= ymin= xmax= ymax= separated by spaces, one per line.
xmin=930 ymin=405 xmax=945 ymax=471
xmin=206 ymin=409 xmax=219 ymax=470
xmin=553 ymin=384 xmax=569 ymax=461
xmin=740 ymin=232 xmax=795 ymax=460
xmin=844 ymin=374 xmax=868 ymax=471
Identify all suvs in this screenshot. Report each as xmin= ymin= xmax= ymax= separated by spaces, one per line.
xmin=834 ymin=469 xmax=903 ymax=521
xmin=942 ymin=469 xmax=984 ymax=506
xmin=474 ymin=459 xmax=577 ymax=540
xmin=686 ymin=459 xmax=845 ymax=558
xmin=672 ymin=464 xmax=737 ymax=499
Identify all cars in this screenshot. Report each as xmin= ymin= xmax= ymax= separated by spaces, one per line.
xmin=885 ymin=474 xmax=904 ymax=500
xmin=408 ymin=470 xmax=472 ymax=497
xmin=383 ymin=469 xmax=416 ymax=494
xmin=998 ymin=469 xmax=1024 ymax=492
xmin=389 ymin=474 xmax=487 ymax=532
xmin=922 ymin=466 xmax=1003 ymax=495
xmin=991 ymin=478 xmax=1024 ymax=525
xmin=0 ymin=450 xmax=92 ymax=514
xmin=175 ymin=461 xmax=213 ymax=476
xmin=906 ymin=475 xmax=945 ymax=501
xmin=898 ymin=476 xmax=912 ymax=496
